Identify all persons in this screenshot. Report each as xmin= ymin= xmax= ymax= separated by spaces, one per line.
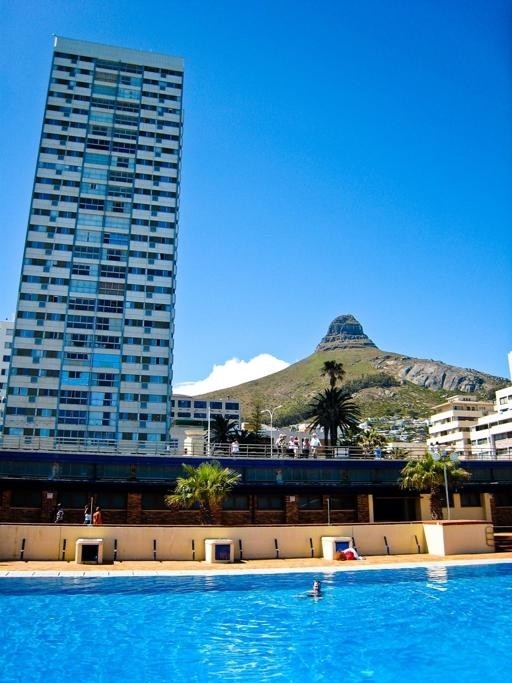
xmin=274 ymin=431 xmax=323 ymax=458
xmin=93 ymin=505 xmax=103 ymax=523
xmin=428 ymin=441 xmax=435 ymax=457
xmin=54 ymin=502 xmax=66 ymax=523
xmin=373 ymin=444 xmax=382 ymax=458
xmin=296 ymin=580 xmax=326 ymax=597
xmin=334 ymin=545 xmax=366 ymax=561
xmin=82 ymin=504 xmax=92 ymax=523
xmin=441 ymin=442 xmax=449 ymax=452
xmin=434 ymin=441 xmax=440 ymax=451
xmin=231 ymin=437 xmax=241 ymax=456
xmin=167 ymin=443 xmax=174 ymax=455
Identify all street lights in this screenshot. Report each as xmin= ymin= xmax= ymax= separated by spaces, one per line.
xmin=1 ymin=395 xmax=8 ymax=403
xmin=431 ymin=448 xmax=459 ymax=520
xmin=259 ymin=403 xmax=283 ymax=458
